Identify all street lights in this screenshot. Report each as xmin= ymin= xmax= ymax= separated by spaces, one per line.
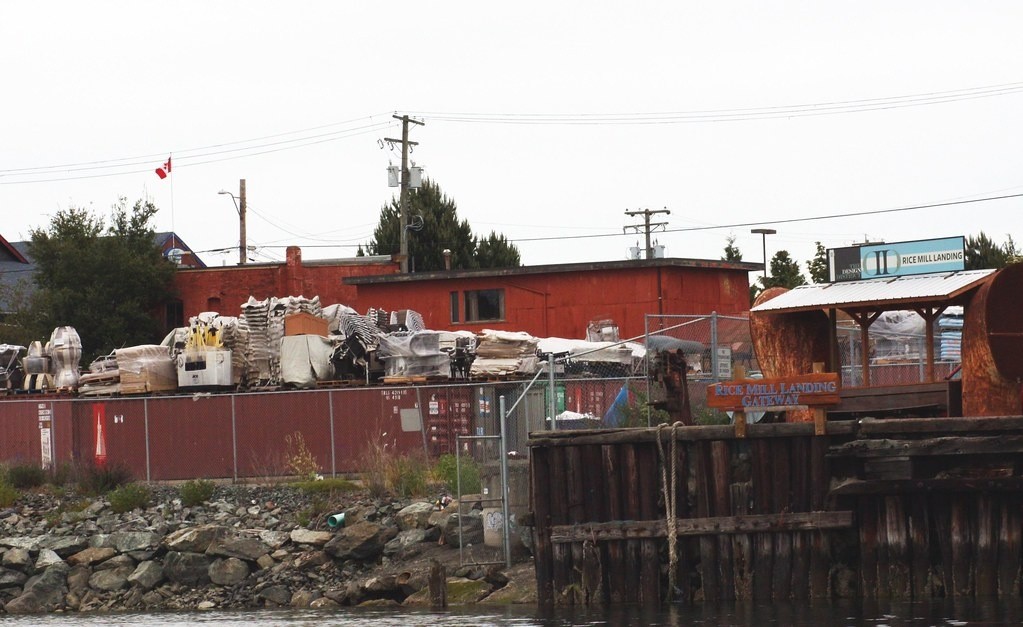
xmin=751 ymin=229 xmax=776 ymax=277
xmin=218 ymin=192 xmax=247 ymax=263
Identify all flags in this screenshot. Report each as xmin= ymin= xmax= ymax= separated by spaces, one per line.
xmin=155 ymin=156 xmax=173 ymax=181
